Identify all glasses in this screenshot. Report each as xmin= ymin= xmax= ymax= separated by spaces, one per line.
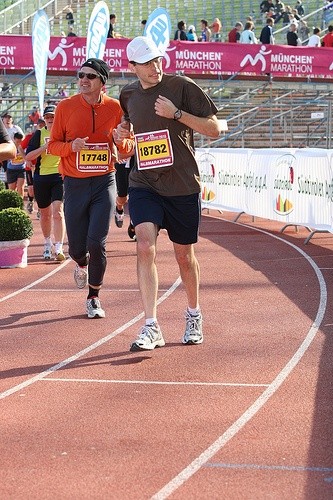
xmin=77 ymin=71 xmax=100 ymax=79
xmin=44 ymin=115 xmax=54 ymax=119
xmin=135 ymin=57 xmax=164 ymax=67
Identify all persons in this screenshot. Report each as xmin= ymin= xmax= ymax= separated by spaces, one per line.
xmin=56 ymin=88 xmax=65 ymax=105
xmin=3 ymin=113 xmax=26 ymax=189
xmin=66 ymin=8 xmax=74 ymax=29
xmin=0 ymin=116 xmax=17 ymax=168
xmin=260 ymin=0 xmax=333 ymax=48
xmin=174 ymin=17 xmax=221 ymax=42
xmin=240 ymin=17 xmax=259 ymax=45
xmin=67 ymin=27 xmax=77 ymax=37
xmin=6 ymin=132 xmax=26 ymax=199
xmin=28 ymin=106 xmax=40 ymax=124
xmin=48 ymin=57 xmax=125 ymax=318
xmin=260 ymin=18 xmax=275 ymax=44
xmin=227 ymin=22 xmax=242 ymax=43
xmin=114 ymin=111 xmax=138 ymax=238
xmin=25 ymin=104 xmax=66 ymax=261
xmin=119 ymin=36 xmax=220 ymax=352
xmin=18 ymin=119 xmax=46 ymax=219
xmin=45 ymin=89 xmax=52 ymax=103
xmin=107 ymin=14 xmax=116 ymax=38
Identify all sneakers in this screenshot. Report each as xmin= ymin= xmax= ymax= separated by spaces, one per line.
xmin=182 ymin=308 xmax=203 ymax=344
xmin=85 ymin=297 xmax=105 ymax=318
xmin=115 ymin=207 xmax=123 ymax=228
xmin=74 ymin=253 xmax=89 ymax=289
xmin=128 ymin=217 xmax=136 ymax=239
xmin=27 ymin=197 xmax=34 ymax=213
xmin=56 ymin=250 xmax=65 ymax=260
xmin=129 ymin=322 xmax=166 ymax=352
xmin=43 ymin=244 xmax=53 ymax=259
xmin=37 ymin=210 xmax=40 ymax=219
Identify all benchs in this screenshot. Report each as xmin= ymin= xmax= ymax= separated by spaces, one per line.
xmin=0 ymin=0 xmax=333 ymax=148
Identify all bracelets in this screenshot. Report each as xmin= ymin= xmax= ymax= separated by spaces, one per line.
xmin=174 ymin=109 xmax=183 ymax=121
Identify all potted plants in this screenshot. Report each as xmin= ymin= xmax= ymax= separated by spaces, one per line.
xmin=0 ymin=207 xmax=34 ymax=269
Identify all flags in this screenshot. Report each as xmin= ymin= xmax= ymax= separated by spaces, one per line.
xmin=85 ymin=1 xmax=110 ymax=61
xmin=144 ymin=8 xmax=170 ymax=62
xmin=31 ymin=10 xmax=51 ymax=115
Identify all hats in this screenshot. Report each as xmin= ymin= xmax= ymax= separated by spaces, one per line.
xmin=126 ymin=35 xmax=165 ymax=64
xmin=3 ymin=113 xmax=13 ymax=117
xmin=42 ymin=106 xmax=56 ymax=115
xmin=80 ymin=58 xmax=110 ymax=85
